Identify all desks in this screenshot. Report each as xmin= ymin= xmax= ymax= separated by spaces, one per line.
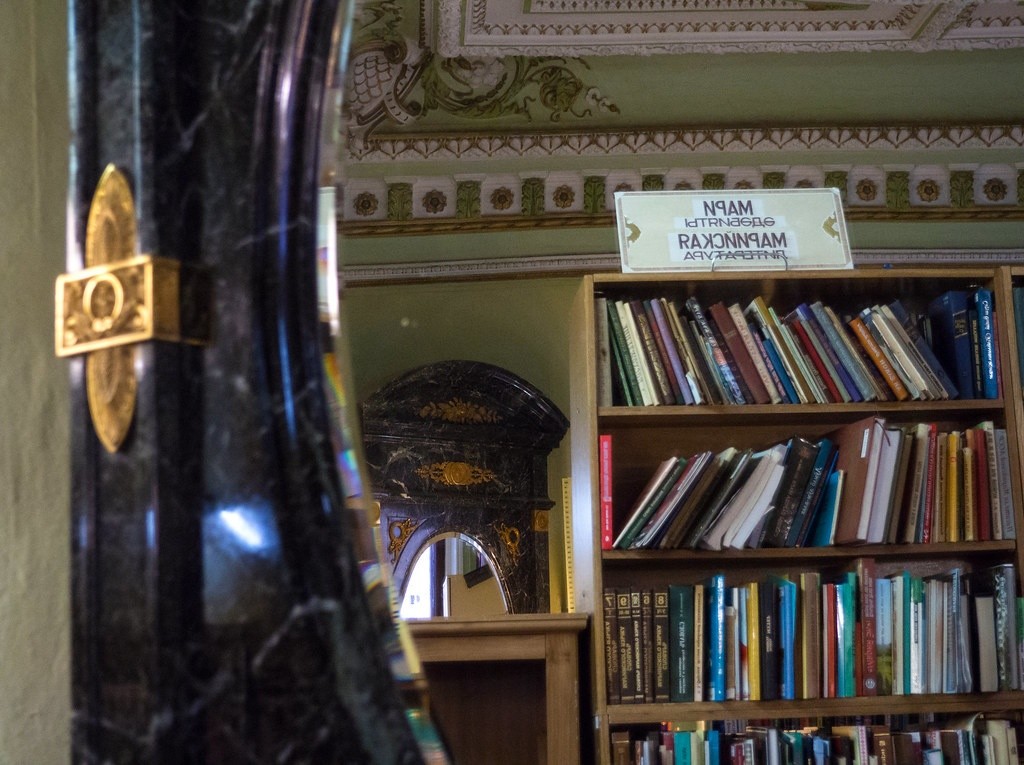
xmin=403 ymin=612 xmax=591 ymax=765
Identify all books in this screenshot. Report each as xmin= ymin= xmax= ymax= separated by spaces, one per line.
xmin=592 ymin=286 xmax=1024 ymax=765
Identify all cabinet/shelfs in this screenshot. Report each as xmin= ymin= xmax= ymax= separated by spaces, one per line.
xmin=567 ymin=266 xmax=1024 ymax=765
